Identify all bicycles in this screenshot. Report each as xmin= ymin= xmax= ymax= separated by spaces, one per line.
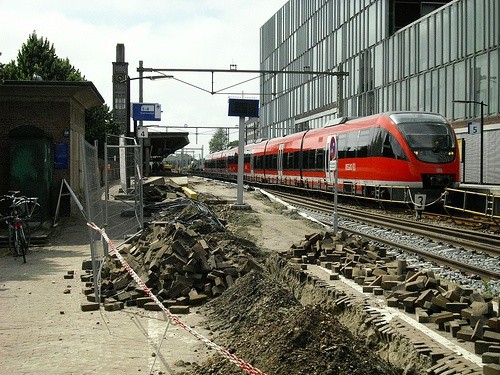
xmin=1 ymin=191 xmax=41 ymax=265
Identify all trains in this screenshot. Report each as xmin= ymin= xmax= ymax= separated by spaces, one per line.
xmin=202 ymin=111 xmax=461 ymax=212
